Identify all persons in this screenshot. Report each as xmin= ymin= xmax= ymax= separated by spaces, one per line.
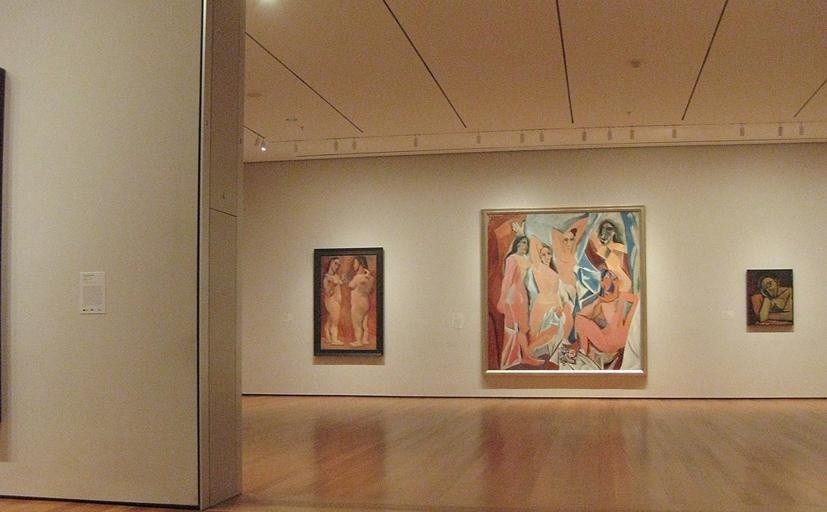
xmin=750 ymin=273 xmax=793 ymax=325
xmin=494 ymin=215 xmax=640 ymax=367
xmin=322 ymin=258 xmax=346 ymax=347
xmin=347 ymin=256 xmax=374 ymax=347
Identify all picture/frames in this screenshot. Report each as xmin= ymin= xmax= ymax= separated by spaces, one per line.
xmin=312 ymin=248 xmax=383 ymax=358
xmin=480 ymin=205 xmax=647 ymax=389
